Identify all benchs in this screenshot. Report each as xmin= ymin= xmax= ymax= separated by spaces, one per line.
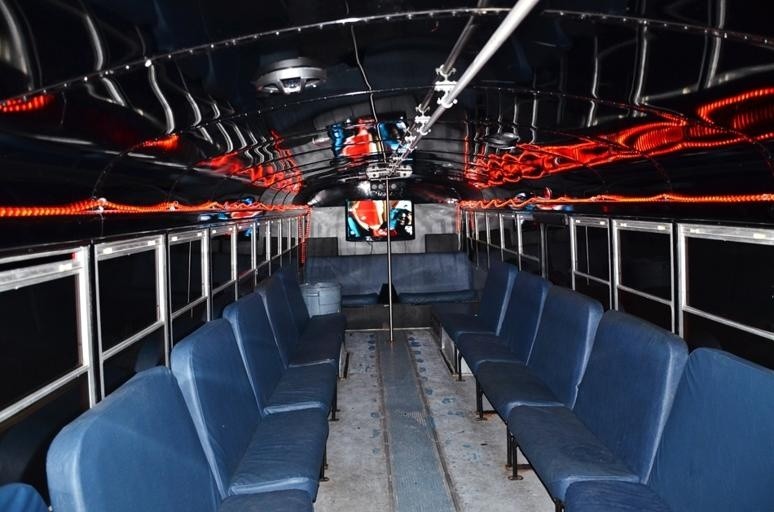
xmin=306 ymin=252 xmax=476 ymax=307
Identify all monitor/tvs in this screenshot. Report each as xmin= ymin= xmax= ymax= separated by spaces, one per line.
xmin=325 ymin=110 xmax=415 ymax=177
xmin=344 ymin=195 xmax=416 ymax=241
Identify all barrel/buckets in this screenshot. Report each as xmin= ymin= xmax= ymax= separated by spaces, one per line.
xmin=301 ymin=282 xmax=341 ymax=317
